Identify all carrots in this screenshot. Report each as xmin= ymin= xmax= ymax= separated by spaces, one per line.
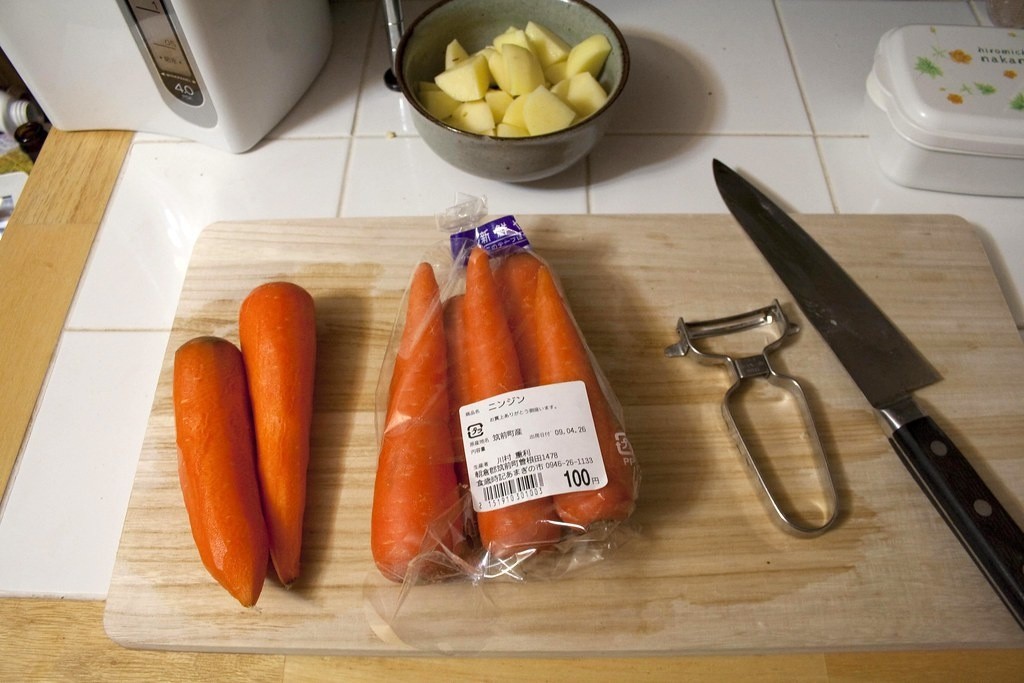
xmin=369 ymin=244 xmax=635 ymax=584
xmin=173 ymin=281 xmax=319 ymax=609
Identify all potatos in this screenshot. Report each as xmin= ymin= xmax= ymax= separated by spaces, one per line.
xmin=418 ymin=19 xmax=611 ymax=139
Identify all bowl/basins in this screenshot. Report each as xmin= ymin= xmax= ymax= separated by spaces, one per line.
xmin=385 ymin=0 xmax=631 ymax=184
xmin=864 ymin=23 xmax=1024 ymax=199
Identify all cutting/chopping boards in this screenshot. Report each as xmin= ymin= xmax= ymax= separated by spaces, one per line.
xmin=105 ymin=213 xmax=1024 ymax=649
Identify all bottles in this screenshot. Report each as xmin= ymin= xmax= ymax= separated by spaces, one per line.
xmin=14 ymin=121 xmax=49 ymax=163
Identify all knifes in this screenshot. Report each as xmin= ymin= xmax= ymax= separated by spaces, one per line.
xmin=712 ymin=158 xmax=1024 ymax=632
